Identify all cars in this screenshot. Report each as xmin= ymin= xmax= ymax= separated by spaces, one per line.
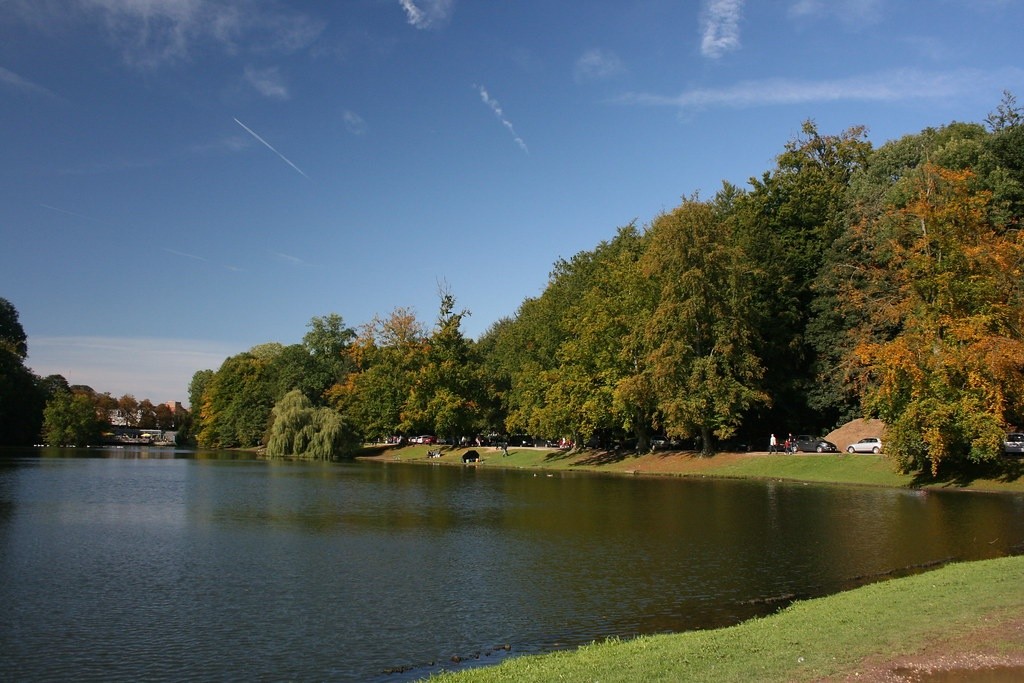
xmin=649 ymin=435 xmax=669 ymax=448
xmin=1001 ymin=433 xmax=1024 ymax=453
xmin=508 ymin=434 xmax=535 ymax=447
xmin=846 ymin=437 xmax=882 ymax=454
xmin=408 ymin=435 xmax=485 ymax=446
xmin=768 ymin=440 xmax=792 ymax=452
xmin=791 ymin=435 xmax=836 ymax=453
xmin=716 ymin=438 xmax=753 ymax=451
xmin=544 ymin=438 xmax=560 ymax=447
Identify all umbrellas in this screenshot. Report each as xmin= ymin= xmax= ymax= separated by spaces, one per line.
xmin=104 ymin=433 xmax=115 ymax=435
xmin=141 ymin=433 xmax=153 ymax=436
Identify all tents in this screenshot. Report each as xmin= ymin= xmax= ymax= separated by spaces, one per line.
xmin=462 ymin=450 xmax=479 ymax=463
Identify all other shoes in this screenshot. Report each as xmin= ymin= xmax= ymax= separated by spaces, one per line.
xmin=784 ymin=452 xmax=786 ymax=455
xmin=789 ymin=452 xmax=793 ymax=455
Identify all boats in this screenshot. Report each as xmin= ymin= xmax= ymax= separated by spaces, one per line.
xmin=150 ymin=435 xmax=173 ymax=445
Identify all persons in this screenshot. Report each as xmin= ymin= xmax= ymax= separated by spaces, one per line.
xmin=385 ymin=436 xmax=397 ymax=444
xmin=475 ymin=435 xmax=483 ymax=447
xmin=557 ymin=438 xmax=574 ymax=448
xmin=502 ymin=442 xmax=508 ymax=457
xmin=428 ymin=435 xmax=434 ymax=446
xmin=769 ymin=434 xmax=778 ymax=454
xmin=489 ymin=434 xmax=496 ymax=447
xmin=458 ymin=436 xmax=472 ymax=447
xmin=427 ymin=450 xmax=441 ymax=455
xmin=784 ymin=434 xmax=795 ymax=455
